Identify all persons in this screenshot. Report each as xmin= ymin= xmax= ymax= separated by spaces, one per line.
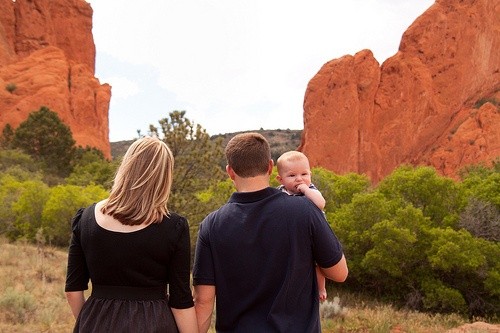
xmin=192 ymin=131 xmax=349 ymax=333
xmin=65 ymin=137 xmax=199 ymax=333
xmin=273 ymin=150 xmax=328 ymax=301
